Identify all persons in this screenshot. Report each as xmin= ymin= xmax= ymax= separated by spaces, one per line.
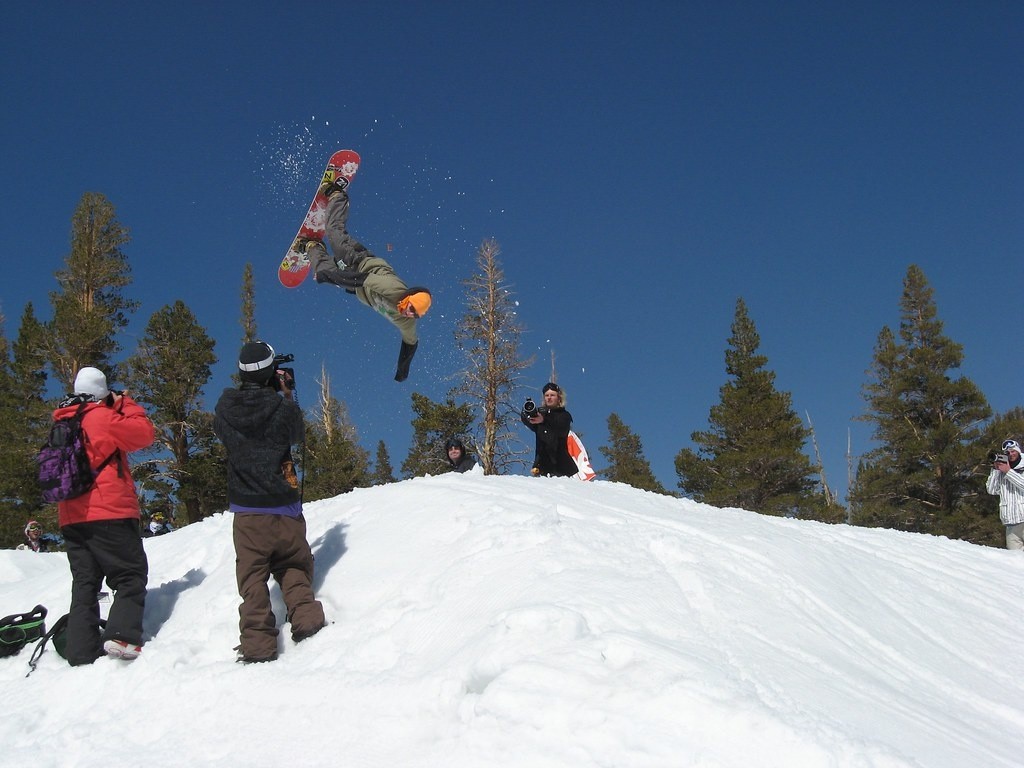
xmin=212 ymin=341 xmax=325 ymax=664
xmin=986 ymin=440 xmax=1024 ymax=550
xmin=445 ymin=438 xmax=476 ymax=473
xmin=143 ymin=513 xmax=171 ymax=538
xmin=16 ymin=521 xmax=51 ymax=552
xmin=520 ymin=383 xmax=579 ymax=478
xmin=52 ymin=366 xmax=155 ymax=666
xmin=293 ymin=183 xmax=432 ymax=382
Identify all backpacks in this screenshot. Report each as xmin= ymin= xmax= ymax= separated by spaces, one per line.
xmin=26 ymin=401 xmax=123 ymax=503
xmin=0 ymin=604 xmax=48 ymax=658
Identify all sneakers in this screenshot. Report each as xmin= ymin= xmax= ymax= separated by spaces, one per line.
xmin=292 ymin=238 xmax=326 ymax=253
xmin=103 ymin=638 xmax=143 ymax=660
xmin=319 ymin=181 xmax=351 ymax=206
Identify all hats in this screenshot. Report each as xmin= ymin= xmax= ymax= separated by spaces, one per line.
xmin=74 ymin=367 xmax=109 ymax=400
xmin=397 ymin=286 xmax=431 ymax=318
xmin=1003 ymin=441 xmax=1021 ymax=454
xmin=149 ymin=507 xmax=162 ymax=518
xmin=25 ymin=521 xmax=43 ymax=540
xmin=236 ymin=339 xmax=276 ymax=383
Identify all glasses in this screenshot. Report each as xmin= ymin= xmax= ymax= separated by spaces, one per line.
xmin=444 ymin=438 xmax=463 ymax=450
xmin=1001 ymin=440 xmax=1016 ymax=450
xmin=542 ymin=382 xmax=561 ymax=395
xmin=28 ymin=525 xmax=41 ymax=531
xmin=409 ymin=305 xmax=420 ymax=319
xmin=153 ymin=515 xmax=165 ymax=520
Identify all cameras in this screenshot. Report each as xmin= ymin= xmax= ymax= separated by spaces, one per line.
xmin=523 ymin=400 xmax=538 ymax=418
xmin=268 ymin=354 xmax=296 ymax=392
xmin=989 ymin=450 xmax=1008 ymax=463
xmin=104 ymin=388 xmax=124 ymax=407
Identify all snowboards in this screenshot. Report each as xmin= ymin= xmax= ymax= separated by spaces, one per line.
xmin=278 ymin=148 xmax=361 ymax=288
